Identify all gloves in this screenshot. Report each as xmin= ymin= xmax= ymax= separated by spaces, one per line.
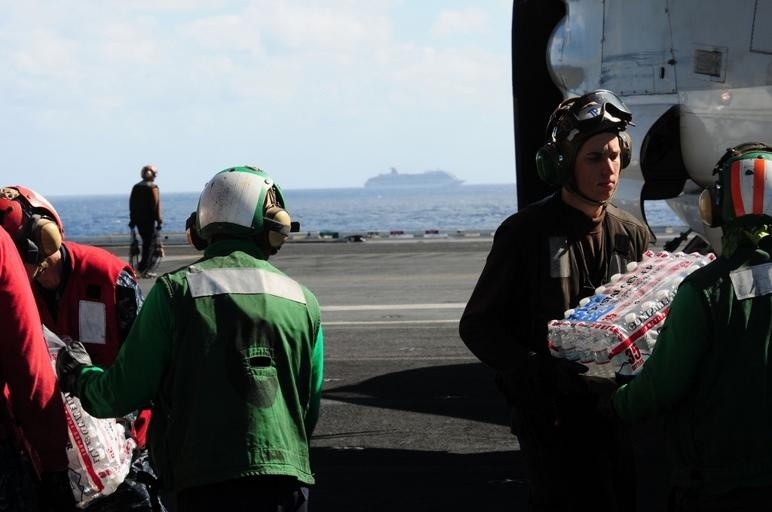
xmin=57 ymin=342 xmax=93 ymax=397
xmin=525 ymin=355 xmax=589 ymax=388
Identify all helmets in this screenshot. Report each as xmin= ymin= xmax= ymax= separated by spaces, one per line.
xmin=186 ymin=166 xmax=292 ymax=255
xmin=142 ymin=166 xmax=157 ymax=180
xmin=536 ymin=96 xmax=631 ymax=184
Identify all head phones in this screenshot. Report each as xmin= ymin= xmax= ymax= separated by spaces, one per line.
xmin=145 ymin=170 xmax=153 ymax=178
xmin=535 ymin=131 xmax=634 ymax=184
xmin=185 ymin=206 xmax=293 ymax=252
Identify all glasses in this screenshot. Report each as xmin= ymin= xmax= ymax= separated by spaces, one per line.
xmin=555 ymin=90 xmax=633 ymax=152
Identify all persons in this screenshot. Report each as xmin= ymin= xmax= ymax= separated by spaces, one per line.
xmin=460 ymin=87 xmax=649 ymax=511
xmin=55 ymin=164 xmax=324 ymax=511
xmin=591 ymin=140 xmax=772 ymax=512
xmin=127 ymin=166 xmax=166 ymax=279
xmin=0 ymin=225 xmax=71 ymax=511
xmin=1 ymin=184 xmax=168 ymax=510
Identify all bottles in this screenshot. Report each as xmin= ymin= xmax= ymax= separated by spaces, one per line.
xmin=546 ymin=248 xmax=716 ymax=378
xmin=60 ymin=392 xmax=138 ymax=507
